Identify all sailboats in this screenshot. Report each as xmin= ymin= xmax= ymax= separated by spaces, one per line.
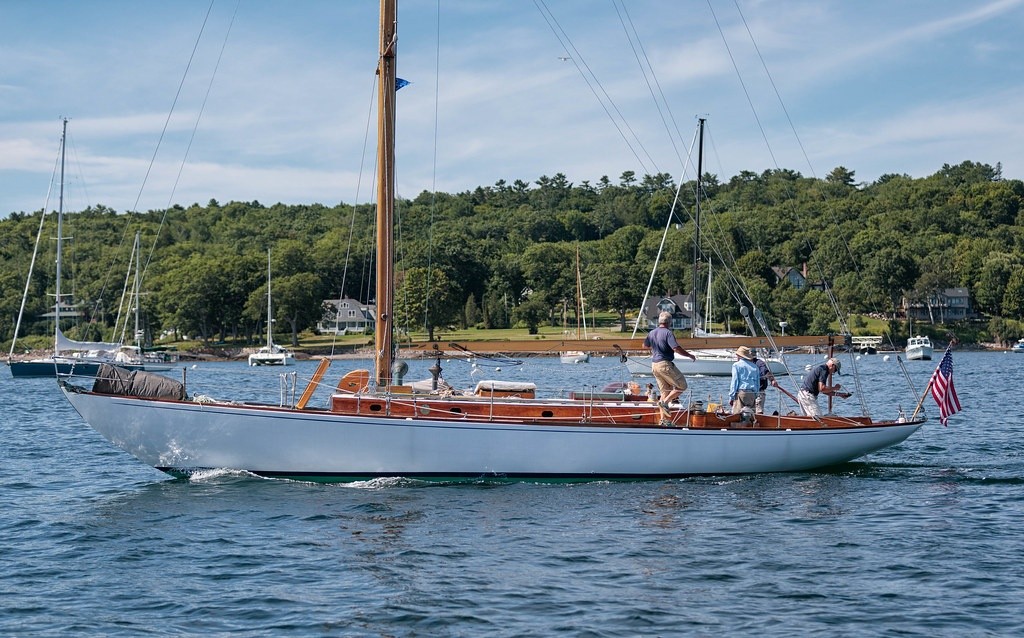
xmin=616 ymin=114 xmax=803 ymax=378
xmin=248 ymin=249 xmax=292 ymax=366
xmin=46 ymin=0 xmax=960 ymax=492
xmin=6 ymin=117 xmax=146 ymax=380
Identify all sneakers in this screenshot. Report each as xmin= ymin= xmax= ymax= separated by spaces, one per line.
xmin=659 ymin=419 xmax=668 ymax=426
xmin=658 ymin=400 xmax=671 ymax=417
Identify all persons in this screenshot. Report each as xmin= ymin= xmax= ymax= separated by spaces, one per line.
xmin=749 ymin=349 xmax=778 ymax=415
xmin=726 ymin=345 xmax=761 ymax=415
xmin=641 ymin=311 xmax=696 ymax=427
xmin=796 ymin=357 xmax=851 ymax=418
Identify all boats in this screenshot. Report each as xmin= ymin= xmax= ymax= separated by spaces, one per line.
xmin=1010 ymin=339 xmax=1024 ymax=353
xmin=904 ymin=336 xmax=935 ymax=360
xmin=857 ymin=340 xmax=877 ymax=355
xmin=560 ymin=349 xmax=590 ymax=365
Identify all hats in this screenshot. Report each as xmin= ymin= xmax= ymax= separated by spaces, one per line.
xmin=749 ymin=348 xmax=757 ymax=358
xmin=736 ymin=346 xmax=753 ymax=360
xmin=831 ymin=358 xmax=841 ymax=375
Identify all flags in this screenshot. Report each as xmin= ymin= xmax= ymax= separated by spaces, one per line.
xmin=929 ymin=346 xmax=962 ymax=426
